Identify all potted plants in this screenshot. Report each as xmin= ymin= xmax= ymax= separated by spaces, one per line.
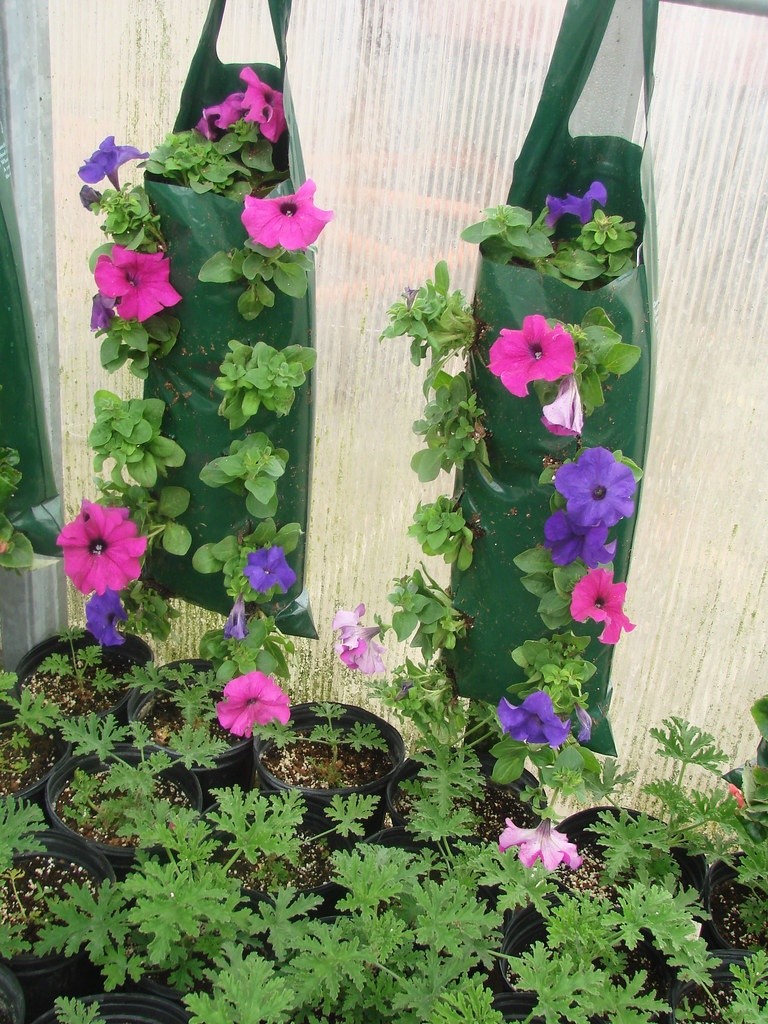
xmin=133 ymin=917 xmax=289 ymax=1003
xmin=127 ymin=659 xmax=252 ymax=805
xmin=374 ymin=871 xmax=514 ymax=972
xmin=549 ymin=806 xmax=709 ymax=919
xmin=669 ymin=949 xmax=768 ymax=1024
xmin=13 ymin=629 xmax=155 ymax=738
xmin=465 ymin=991 xmax=606 ymax=1024
xmin=252 ymin=702 xmax=405 ymax=826
xmin=356 ymin=826 xmax=468 ymax=901
xmin=497 ymin=892 xmax=679 ymax=1021
xmin=34 ymin=994 xmax=192 ymax=1024
xmin=45 ymin=742 xmax=204 ymax=876
xmin=186 ymin=789 xmax=363 ymax=918
xmin=0 ymin=961 xmax=26 ymax=1024
xmin=706 ymin=852 xmax=768 ymax=950
xmin=0 ymin=827 xmax=118 ymax=1005
xmin=386 ymin=746 xmax=549 ymax=849
xmin=0 ymin=692 xmax=72 ymax=805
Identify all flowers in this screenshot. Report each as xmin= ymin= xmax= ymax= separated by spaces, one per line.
xmin=331 ymin=178 xmax=645 ymax=872
xmin=55 ymin=66 xmax=333 ymax=741
